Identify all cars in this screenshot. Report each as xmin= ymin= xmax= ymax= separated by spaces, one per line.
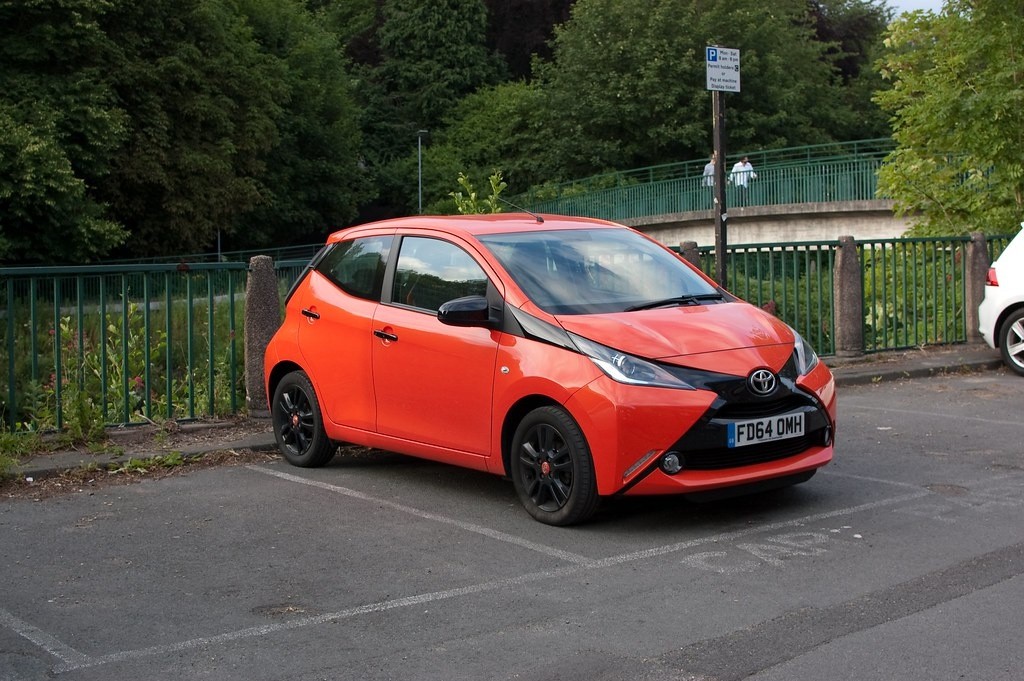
xmin=977 ymin=220 xmax=1024 ymax=376
xmin=263 ymin=212 xmax=840 ymax=528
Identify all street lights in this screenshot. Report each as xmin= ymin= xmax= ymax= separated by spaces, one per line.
xmin=416 ymin=129 xmax=429 ymax=214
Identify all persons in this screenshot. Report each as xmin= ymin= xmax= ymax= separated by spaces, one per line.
xmin=702 ymin=155 xmax=717 ymax=209
xmin=727 ymin=155 xmax=759 ymax=206
